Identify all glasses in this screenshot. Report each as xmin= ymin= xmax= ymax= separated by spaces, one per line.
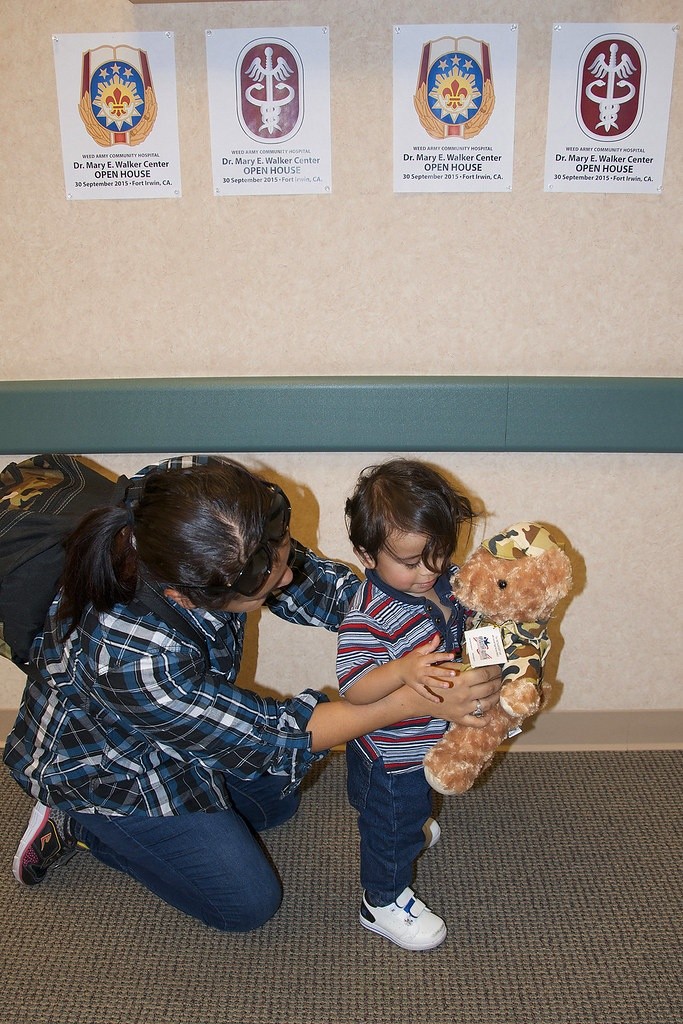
xmin=159 ymin=476 xmax=292 ymax=596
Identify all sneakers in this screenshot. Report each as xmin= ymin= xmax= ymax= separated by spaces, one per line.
xmin=360 ymin=816 xmax=447 ymax=951
xmin=11 ymin=801 xmax=75 ymax=888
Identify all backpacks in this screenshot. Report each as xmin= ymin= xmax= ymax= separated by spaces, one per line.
xmin=0 ymin=452 xmax=135 ymax=682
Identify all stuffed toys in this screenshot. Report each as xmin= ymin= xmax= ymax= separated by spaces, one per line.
xmin=422 ymin=519 xmax=575 ymax=798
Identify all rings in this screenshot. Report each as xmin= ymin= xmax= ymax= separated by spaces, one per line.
xmin=473 ymin=698 xmax=484 ymax=719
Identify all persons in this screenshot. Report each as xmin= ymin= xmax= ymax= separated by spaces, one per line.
xmin=327 ymin=456 xmax=504 ymax=956
xmin=0 ymin=455 xmax=506 ymax=937
